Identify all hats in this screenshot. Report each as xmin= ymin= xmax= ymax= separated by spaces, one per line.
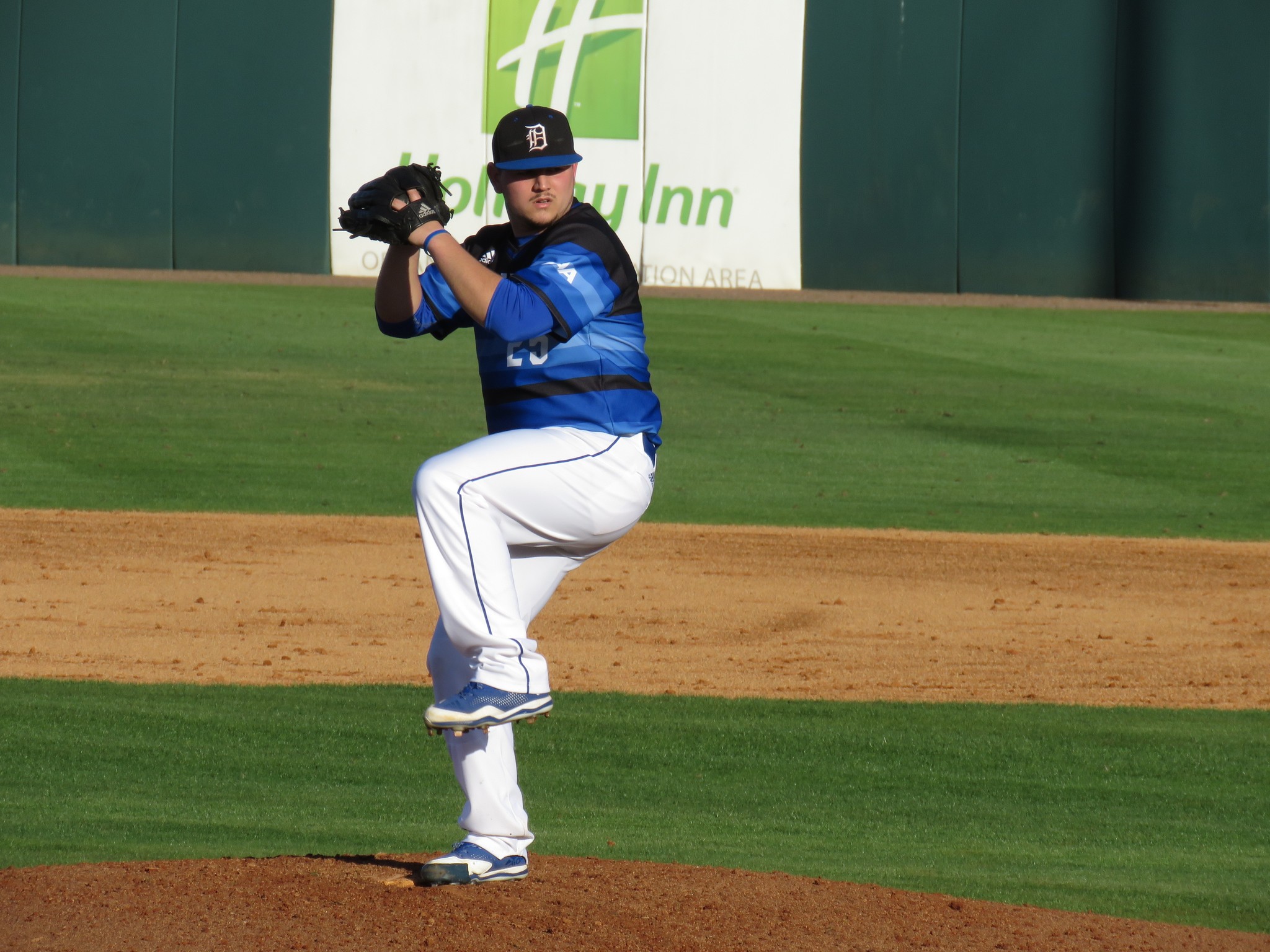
xmin=492 ymin=104 xmax=582 ymax=171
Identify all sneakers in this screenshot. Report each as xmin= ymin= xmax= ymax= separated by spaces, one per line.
xmin=422 ymin=681 xmax=552 ymax=738
xmin=421 ymin=841 xmax=529 ymax=887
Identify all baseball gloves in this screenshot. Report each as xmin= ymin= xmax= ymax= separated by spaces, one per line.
xmin=338 ymin=164 xmax=451 ymax=243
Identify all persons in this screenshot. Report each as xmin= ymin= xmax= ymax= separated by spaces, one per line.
xmin=332 ymin=104 xmax=662 ymax=889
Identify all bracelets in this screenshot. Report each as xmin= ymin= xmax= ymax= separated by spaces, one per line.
xmin=422 ymin=230 xmax=452 ymax=255
